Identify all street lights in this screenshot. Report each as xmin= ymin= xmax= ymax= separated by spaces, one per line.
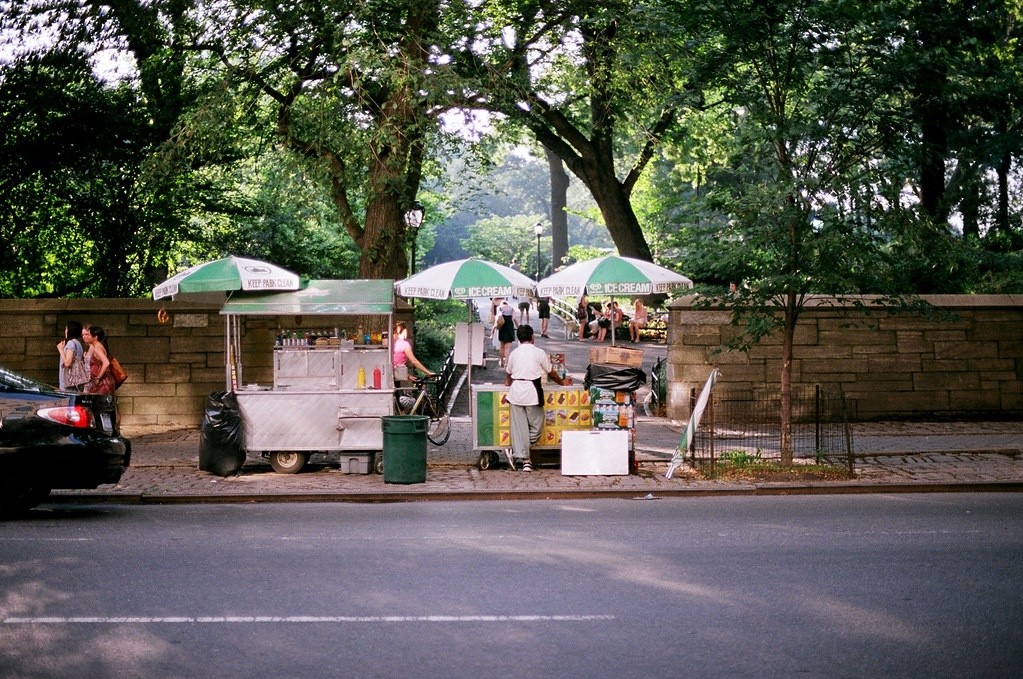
xmin=534 ymin=222 xmax=544 ymax=283
xmin=403 ymin=199 xmax=426 ymax=369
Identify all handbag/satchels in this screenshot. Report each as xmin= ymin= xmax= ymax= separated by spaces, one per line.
xmin=598 ymin=316 xmax=611 ymax=327
xmin=61 ymin=340 xmax=89 ymax=387
xmin=494 ymin=307 xmax=505 ymax=330
xmin=109 ymin=357 xmax=128 ymax=389
xmin=488 ymin=312 xmax=495 ymax=324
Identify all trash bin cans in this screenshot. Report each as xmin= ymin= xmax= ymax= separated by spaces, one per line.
xmin=382 ymin=415 xmax=428 ymax=484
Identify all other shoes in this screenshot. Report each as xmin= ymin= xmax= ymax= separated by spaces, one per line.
xmin=483 ymin=366 xmax=487 ymax=370
xmin=541 ymin=333 xmax=548 ymax=338
xmin=523 ymin=462 xmax=533 ymax=471
xmin=505 ymin=448 xmax=516 ymax=471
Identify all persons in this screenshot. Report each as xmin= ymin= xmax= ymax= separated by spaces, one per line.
xmin=505 ymin=325 xmax=572 ymax=473
xmin=510 ymin=259 xmax=520 ymax=299
xmin=488 ymin=297 xmax=519 ymax=367
xmin=478 ymin=327 xmax=493 ymax=371
xmin=392 ymin=322 xmax=435 ymax=415
xmin=57 ymin=321 xmax=115 ymax=397
xmin=628 ymin=299 xmax=648 ymax=343
xmin=577 ymin=296 xmax=622 ymax=344
xmin=537 ymin=296 xmax=556 ymax=338
xmin=517 ymin=296 xmax=533 ymax=326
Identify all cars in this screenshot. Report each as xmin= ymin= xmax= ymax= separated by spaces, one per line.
xmin=0 ymin=367 xmax=130 ymax=516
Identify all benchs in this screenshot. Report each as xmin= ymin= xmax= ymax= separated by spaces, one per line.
xmin=608 ymin=313 xmax=669 ymax=342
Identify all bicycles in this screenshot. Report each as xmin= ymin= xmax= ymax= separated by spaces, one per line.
xmin=393 ymin=373 xmax=451 ymax=446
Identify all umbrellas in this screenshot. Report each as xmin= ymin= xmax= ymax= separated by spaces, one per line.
xmin=666 ymin=368 xmax=717 ymax=480
xmin=538 ymin=254 xmax=692 ymax=347
xmin=150 ymin=255 xmax=306 ymax=363
xmin=392 ymin=256 xmax=536 ymax=417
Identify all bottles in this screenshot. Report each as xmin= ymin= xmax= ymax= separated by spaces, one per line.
xmin=594 ymin=405 xmax=635 ymax=451
xmin=374 ymin=366 xmax=382 ymax=388
xmin=547 ymin=363 xmax=566 ymax=383
xmin=276 ymin=326 xmax=389 ymax=349
xmin=357 ymin=366 xmax=366 ymax=389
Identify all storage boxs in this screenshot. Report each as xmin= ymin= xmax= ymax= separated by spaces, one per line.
xmin=591 ymin=346 xmax=642 ymax=367
xmin=560 ymin=430 xmax=628 ymax=475
xmin=339 ymin=451 xmax=370 ymax=472
